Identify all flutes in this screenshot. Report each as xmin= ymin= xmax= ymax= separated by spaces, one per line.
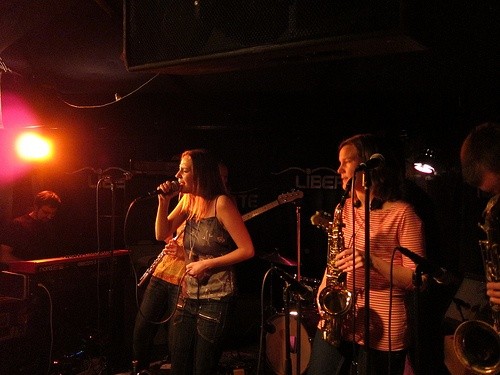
xmin=137 ymin=225 xmax=186 ymax=288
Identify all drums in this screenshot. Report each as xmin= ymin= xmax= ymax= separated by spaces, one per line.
xmin=284 ymin=274 xmax=320 ymax=314
xmin=262 ymin=313 xmax=312 ymax=375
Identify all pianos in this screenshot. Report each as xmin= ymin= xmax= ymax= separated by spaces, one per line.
xmin=6 ymin=249 xmax=133 ymax=359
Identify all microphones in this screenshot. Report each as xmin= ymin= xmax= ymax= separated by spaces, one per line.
xmin=274 ymin=267 xmax=315 ymax=300
xmin=148 ymin=180 xmax=179 ymax=198
xmin=355 ymin=153 xmax=386 ymax=172
xmin=397 ymin=247 xmax=430 ymax=265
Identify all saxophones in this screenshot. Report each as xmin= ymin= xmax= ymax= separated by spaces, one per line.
xmin=317 ymin=176 xmax=356 ymax=348
xmin=453 ymin=193 xmax=500 ymax=375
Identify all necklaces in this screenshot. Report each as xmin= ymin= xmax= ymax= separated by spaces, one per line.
xmin=189 ymin=196 xmax=206 ymax=260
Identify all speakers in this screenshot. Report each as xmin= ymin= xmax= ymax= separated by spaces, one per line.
xmin=123 ymin=0 xmax=437 ymax=76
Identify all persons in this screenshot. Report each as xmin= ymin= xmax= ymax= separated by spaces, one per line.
xmin=461 ymin=121 xmax=500 ymax=334
xmin=302 ymin=132 xmax=427 ymax=375
xmin=0 ymin=191 xmax=65 ymax=355
xmin=133 ymin=149 xmax=255 ymax=375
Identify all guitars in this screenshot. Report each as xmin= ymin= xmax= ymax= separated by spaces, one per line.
xmin=241 ymin=188 xmax=304 ymax=223
xmin=311 ymin=211 xmax=333 ymax=231
